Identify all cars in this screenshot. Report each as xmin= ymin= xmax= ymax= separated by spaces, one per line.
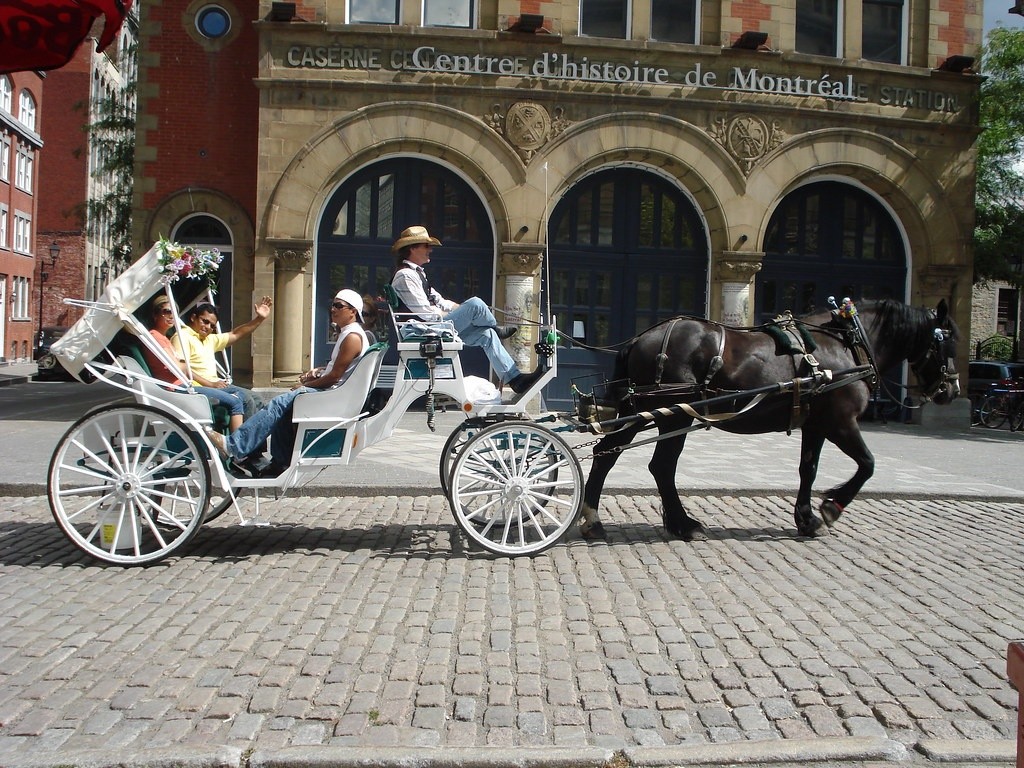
xmin=32 ymin=326 xmax=75 ymax=383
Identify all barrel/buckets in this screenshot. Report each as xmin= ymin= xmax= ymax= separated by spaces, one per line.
xmin=97 ymin=504 xmax=143 ymax=550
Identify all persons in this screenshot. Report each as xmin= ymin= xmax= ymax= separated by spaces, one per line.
xmin=202 ymin=288 xmax=371 ymax=461
xmin=142 ymin=296 xmax=262 ymax=478
xmin=390 ymin=226 xmax=543 ymax=393
xmin=170 ymin=296 xmax=273 ymax=470
xmin=261 ymin=297 xmax=378 ymax=475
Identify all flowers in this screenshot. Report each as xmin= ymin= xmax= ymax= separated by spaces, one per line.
xmin=155 ymin=231 xmax=227 ymax=295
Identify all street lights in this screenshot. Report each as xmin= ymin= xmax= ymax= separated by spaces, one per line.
xmin=37 ymin=240 xmax=61 ymax=359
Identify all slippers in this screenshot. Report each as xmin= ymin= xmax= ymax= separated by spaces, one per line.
xmin=204 ymin=426 xmax=229 ymax=461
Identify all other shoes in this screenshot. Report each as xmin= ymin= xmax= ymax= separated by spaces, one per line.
xmin=231 ymin=456 xmax=263 ymax=478
xmin=253 ymin=457 xmax=272 ymax=471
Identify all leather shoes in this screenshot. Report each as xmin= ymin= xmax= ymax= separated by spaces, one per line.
xmin=510 ymin=364 xmax=543 ymax=394
xmin=494 ymin=325 xmax=517 ymax=340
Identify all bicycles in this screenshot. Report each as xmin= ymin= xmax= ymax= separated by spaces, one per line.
xmin=968 ymin=382 xmax=1024 ymax=433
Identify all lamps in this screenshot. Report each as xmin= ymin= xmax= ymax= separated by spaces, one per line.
xmin=518 ymin=13 xmax=545 ymax=32
xmin=272 ymin=1 xmax=295 ymax=20
xmin=740 ymin=30 xmax=770 ymax=48
xmin=946 ymin=54 xmax=976 ymax=72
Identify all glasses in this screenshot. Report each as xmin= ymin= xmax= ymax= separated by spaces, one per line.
xmin=198 ymin=316 xmax=217 ymax=330
xmin=160 ymin=308 xmax=172 ymax=316
xmin=332 ymin=302 xmax=353 ymax=310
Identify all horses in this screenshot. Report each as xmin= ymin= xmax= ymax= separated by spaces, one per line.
xmin=577 ymin=298 xmax=960 ymax=542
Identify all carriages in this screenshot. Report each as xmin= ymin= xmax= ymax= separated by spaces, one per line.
xmin=50 ymin=237 xmax=963 ymax=569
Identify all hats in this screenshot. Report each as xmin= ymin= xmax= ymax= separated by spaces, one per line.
xmin=335 ymin=289 xmax=365 ymax=324
xmin=391 ymin=226 xmax=442 ymax=254
xmin=150 ymin=295 xmax=179 ymax=317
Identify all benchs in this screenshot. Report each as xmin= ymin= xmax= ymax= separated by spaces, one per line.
xmin=382 ymin=283 xmax=465 ymax=349
xmin=291 ymin=342 xmax=388 ymax=422
xmin=126 ymin=342 xmax=233 ymax=427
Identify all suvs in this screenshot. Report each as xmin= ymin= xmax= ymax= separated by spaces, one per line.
xmin=968 ymin=360 xmax=1024 ymax=395
